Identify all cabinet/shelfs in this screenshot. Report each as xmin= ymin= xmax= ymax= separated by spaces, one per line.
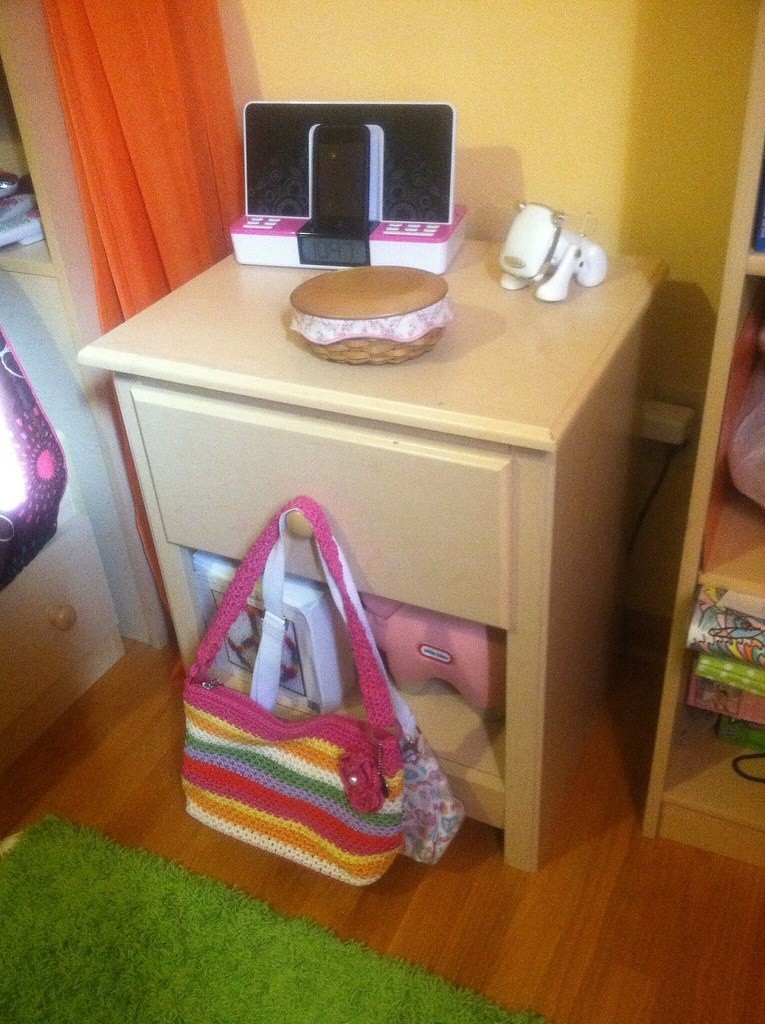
xmin=0 ymin=0 xmax=178 ymax=648
xmin=76 ymin=239 xmax=653 ymax=874
xmin=0 ymin=475 xmax=128 ymax=777
xmin=644 ymin=6 xmax=765 ymax=871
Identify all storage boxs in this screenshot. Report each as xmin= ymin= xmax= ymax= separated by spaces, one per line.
xmin=187 ymin=549 xmax=356 ymax=715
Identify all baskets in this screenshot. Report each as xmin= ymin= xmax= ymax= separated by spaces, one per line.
xmin=291 ymin=266 xmax=449 ymax=364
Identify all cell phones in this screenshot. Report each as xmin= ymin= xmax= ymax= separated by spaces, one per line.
xmin=312 ymin=124 xmax=370 ymax=238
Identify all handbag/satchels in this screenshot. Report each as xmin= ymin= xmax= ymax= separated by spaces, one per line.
xmin=176 ymin=498 xmax=464 ymax=887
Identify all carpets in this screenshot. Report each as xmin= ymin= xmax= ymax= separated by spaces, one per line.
xmin=0 ymin=814 xmax=551 ymax=1024
